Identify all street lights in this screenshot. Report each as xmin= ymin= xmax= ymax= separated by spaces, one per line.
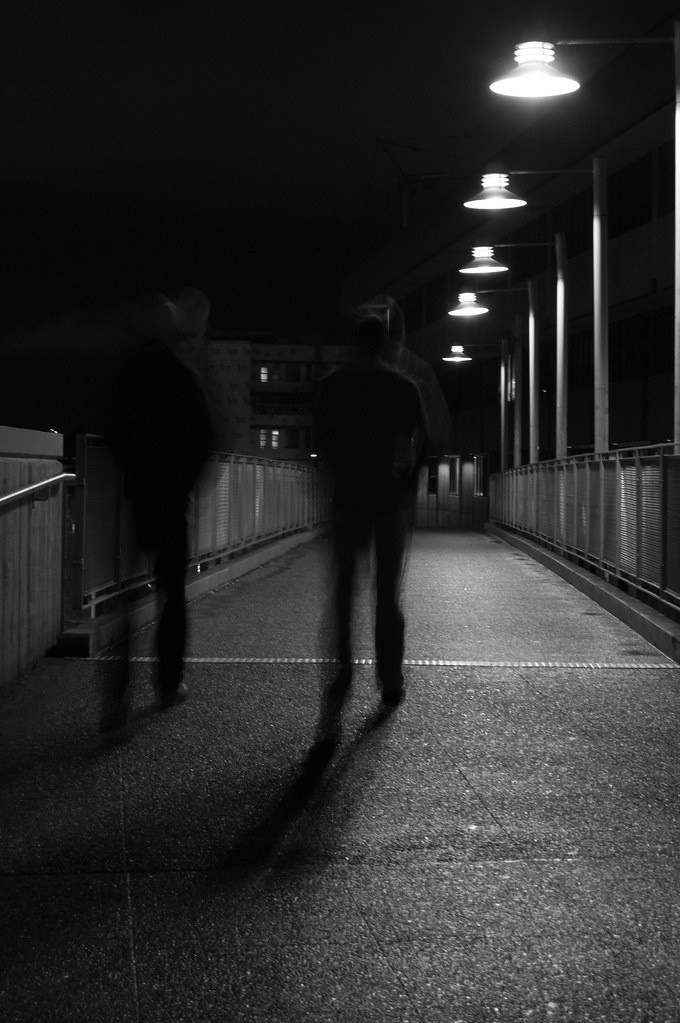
xmin=460 ymin=151 xmax=610 ymax=461
xmin=447 ymin=280 xmax=540 ymax=465
xmin=487 ymin=23 xmax=679 ymax=459
xmin=458 ymin=230 xmax=567 ymax=461
xmin=441 ymin=312 xmax=522 ymax=469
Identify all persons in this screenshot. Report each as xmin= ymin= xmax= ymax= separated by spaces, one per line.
xmin=312 ymin=296 xmax=432 ymax=708
xmin=99 ymin=294 xmax=214 ymax=736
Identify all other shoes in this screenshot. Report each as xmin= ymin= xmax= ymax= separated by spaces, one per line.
xmin=100 ymin=702 xmax=130 ymax=730
xmin=159 ymin=682 xmax=188 ymax=707
xmin=331 ymin=669 xmax=350 ymax=696
xmin=378 ymin=686 xmax=405 ymax=707
